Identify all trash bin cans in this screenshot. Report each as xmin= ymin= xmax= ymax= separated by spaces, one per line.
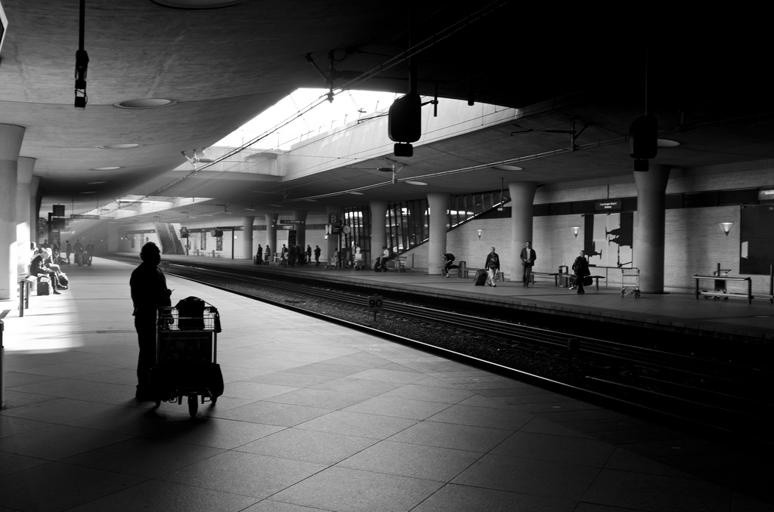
xmin=558 ymin=265 xmax=568 ymax=287
xmin=37 ymin=276 xmax=50 ymax=296
xmin=458 ymin=260 xmax=466 ymax=277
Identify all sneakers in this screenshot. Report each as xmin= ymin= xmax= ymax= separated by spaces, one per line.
xmin=58 ymin=285 xmax=67 ymax=290
xmin=487 ymin=282 xmax=496 ymax=287
xmin=54 ymin=290 xmax=61 ymax=294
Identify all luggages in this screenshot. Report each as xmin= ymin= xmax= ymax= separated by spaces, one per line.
xmin=474 ymin=269 xmax=488 ymax=285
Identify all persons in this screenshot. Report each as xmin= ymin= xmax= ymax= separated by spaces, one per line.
xmin=280 ymin=241 xmax=304 ymax=267
xmin=572 ymin=250 xmax=589 ymax=294
xmin=306 ymin=245 xmax=311 ymax=263
xmin=442 ymin=254 xmax=458 ymax=278
xmin=264 ymin=245 xmax=270 ymax=260
xmin=521 ymin=240 xmax=536 ymax=288
xmin=32 ymin=237 xmax=105 ymax=294
xmin=257 ymin=244 xmax=263 ymax=264
xmin=374 ymin=246 xmax=390 ymax=272
xmin=330 ymin=243 xmax=363 ymax=270
xmin=314 ymin=245 xmax=321 ymax=265
xmin=484 ymin=246 xmax=500 ymax=287
xmin=129 ymin=242 xmax=173 ymax=401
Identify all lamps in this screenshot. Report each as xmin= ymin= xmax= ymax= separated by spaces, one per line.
xmin=572 ymin=227 xmax=580 ymax=238
xmin=719 ymin=221 xmax=734 ymax=235
xmin=477 ymin=230 xmax=482 ymax=237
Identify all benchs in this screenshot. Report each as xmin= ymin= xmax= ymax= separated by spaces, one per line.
xmin=440 ymin=263 xmax=485 ymax=278
xmin=563 ymin=274 xmax=607 ymax=290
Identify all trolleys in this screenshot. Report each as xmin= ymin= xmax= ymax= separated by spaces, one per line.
xmin=151 ymin=296 xmax=224 ymax=420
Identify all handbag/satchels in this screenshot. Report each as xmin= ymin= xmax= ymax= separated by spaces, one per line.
xmin=59 ymin=274 xmax=68 ymax=286
